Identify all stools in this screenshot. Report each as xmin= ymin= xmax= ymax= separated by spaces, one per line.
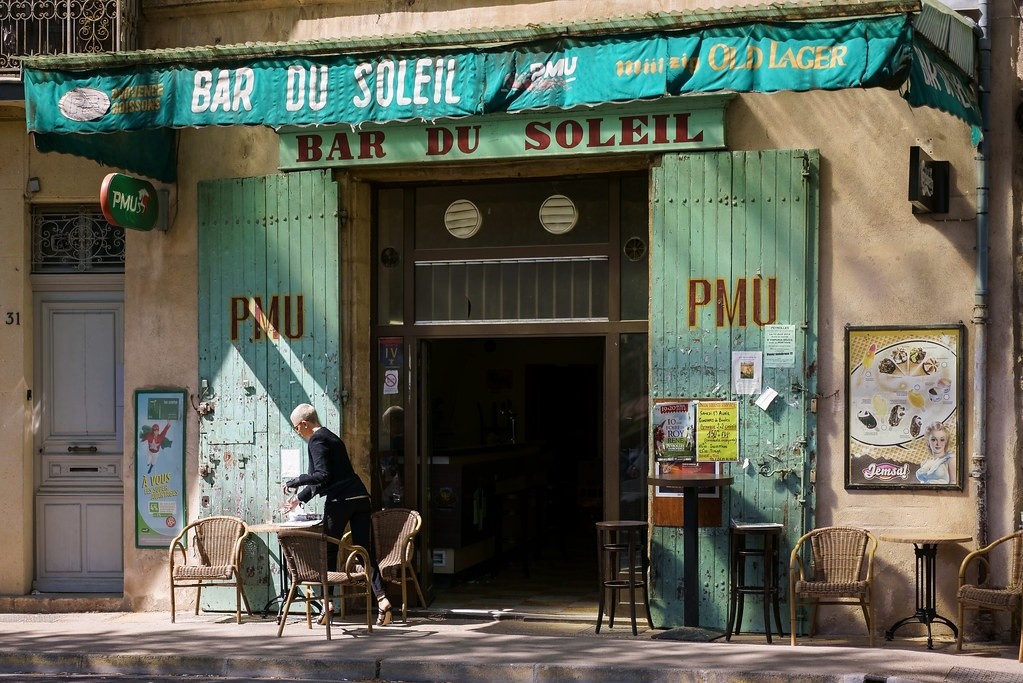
xmin=539 ymin=481 xmax=581 ymax=562
xmin=725 ymin=523 xmax=784 ymax=645
xmin=595 ymin=521 xmax=655 ymax=637
xmin=494 ymin=490 xmax=547 ymax=579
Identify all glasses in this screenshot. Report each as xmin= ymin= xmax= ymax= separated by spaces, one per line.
xmin=293 ymin=420 xmax=309 ymax=434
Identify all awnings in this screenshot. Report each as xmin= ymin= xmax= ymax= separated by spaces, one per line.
xmin=22 ymin=0 xmax=986 ymax=184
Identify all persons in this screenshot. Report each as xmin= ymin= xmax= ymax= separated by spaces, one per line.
xmin=916 ymin=422 xmax=956 ymax=484
xmin=382 ymin=405 xmax=406 ymax=489
xmin=279 ymin=404 xmax=392 ymax=626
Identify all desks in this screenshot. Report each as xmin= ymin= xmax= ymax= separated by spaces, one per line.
xmin=880 ymin=532 xmax=973 ymax=650
xmin=647 ymin=473 xmax=734 ymax=643
xmin=247 ymin=520 xmax=324 ymax=626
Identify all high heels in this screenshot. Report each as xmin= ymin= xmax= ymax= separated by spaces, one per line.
xmin=376 ymin=597 xmax=393 ymax=626
xmin=316 ymin=600 xmax=335 ymax=626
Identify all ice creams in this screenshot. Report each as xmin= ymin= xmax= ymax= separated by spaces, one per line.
xmin=878 ymin=347 xmax=939 ymax=377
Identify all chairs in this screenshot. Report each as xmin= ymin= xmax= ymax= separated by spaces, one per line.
xmin=956 ymin=530 xmax=1023 ymax=662
xmin=277 ymin=531 xmax=373 ymax=641
xmin=339 ymin=507 xmax=428 ymax=624
xmin=169 ymin=515 xmax=250 ymax=624
xmin=789 ymin=525 xmax=878 ymax=646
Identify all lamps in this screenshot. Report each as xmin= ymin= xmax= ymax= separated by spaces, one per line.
xmin=29 ymin=177 xmax=41 ymax=193
